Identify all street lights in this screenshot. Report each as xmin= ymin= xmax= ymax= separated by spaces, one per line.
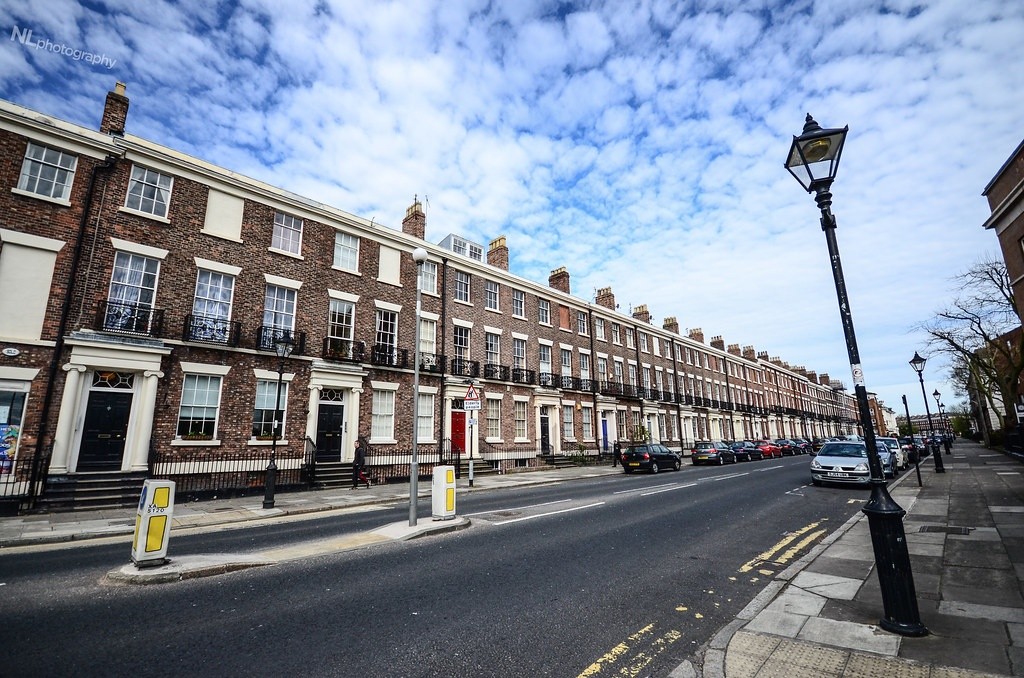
xmin=260 ymin=330 xmax=297 ymax=509
xmin=406 ymin=247 xmax=429 ymax=526
xmin=933 ymin=389 xmax=957 ymax=455
xmin=908 ymin=351 xmax=947 ymax=473
xmin=785 ymin=110 xmax=928 ymax=637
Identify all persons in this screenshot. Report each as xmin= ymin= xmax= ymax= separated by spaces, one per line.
xmin=612 ymin=440 xmax=624 ymax=467
xmin=349 ymin=440 xmax=371 ymax=490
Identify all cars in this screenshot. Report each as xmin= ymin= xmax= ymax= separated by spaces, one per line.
xmin=793 ymin=438 xmax=814 ymax=453
xmin=691 ymin=441 xmax=737 ymax=465
xmin=751 ymin=440 xmax=783 ymax=458
xmin=729 ymin=441 xmax=764 ymax=461
xmin=809 ymin=436 xmax=944 ymax=486
xmin=774 ymin=439 xmax=802 ymax=455
xmin=620 ymin=443 xmax=682 ymax=475
